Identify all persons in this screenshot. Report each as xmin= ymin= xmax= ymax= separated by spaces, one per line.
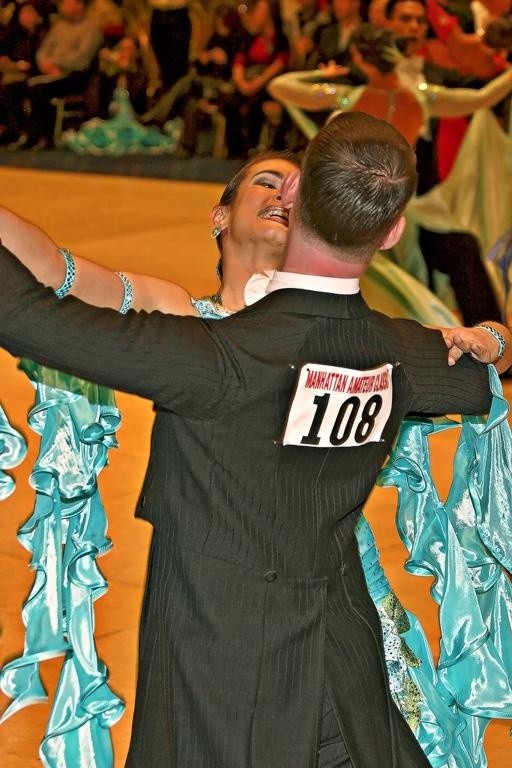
xmin=0 ymin=0 xmax=511 ymax=384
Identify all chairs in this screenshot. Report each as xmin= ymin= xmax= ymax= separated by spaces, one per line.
xmin=51 ymin=67 xmax=103 ymax=138
